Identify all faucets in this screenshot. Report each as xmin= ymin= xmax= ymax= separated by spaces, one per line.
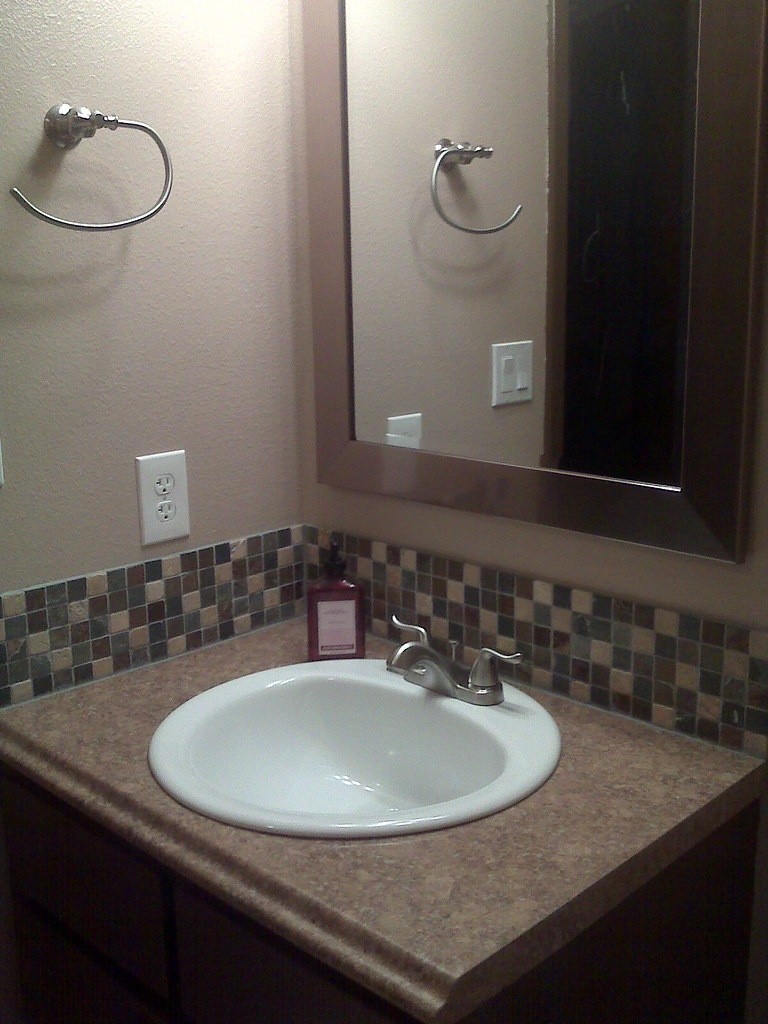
xmin=385 ymin=640 xmax=470 ymax=698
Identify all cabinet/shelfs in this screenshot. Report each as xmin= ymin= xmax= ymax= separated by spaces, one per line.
xmin=0 ymin=751 xmax=757 ymax=1024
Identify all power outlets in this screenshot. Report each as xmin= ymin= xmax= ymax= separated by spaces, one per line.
xmin=136 ymin=450 xmax=191 ymax=546
xmin=388 ymin=414 xmax=423 ymax=450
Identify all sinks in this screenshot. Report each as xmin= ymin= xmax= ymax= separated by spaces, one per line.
xmin=147 ymin=657 xmax=563 ymax=841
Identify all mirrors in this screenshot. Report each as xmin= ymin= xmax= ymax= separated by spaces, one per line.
xmin=301 ymin=0 xmax=768 ymax=565
